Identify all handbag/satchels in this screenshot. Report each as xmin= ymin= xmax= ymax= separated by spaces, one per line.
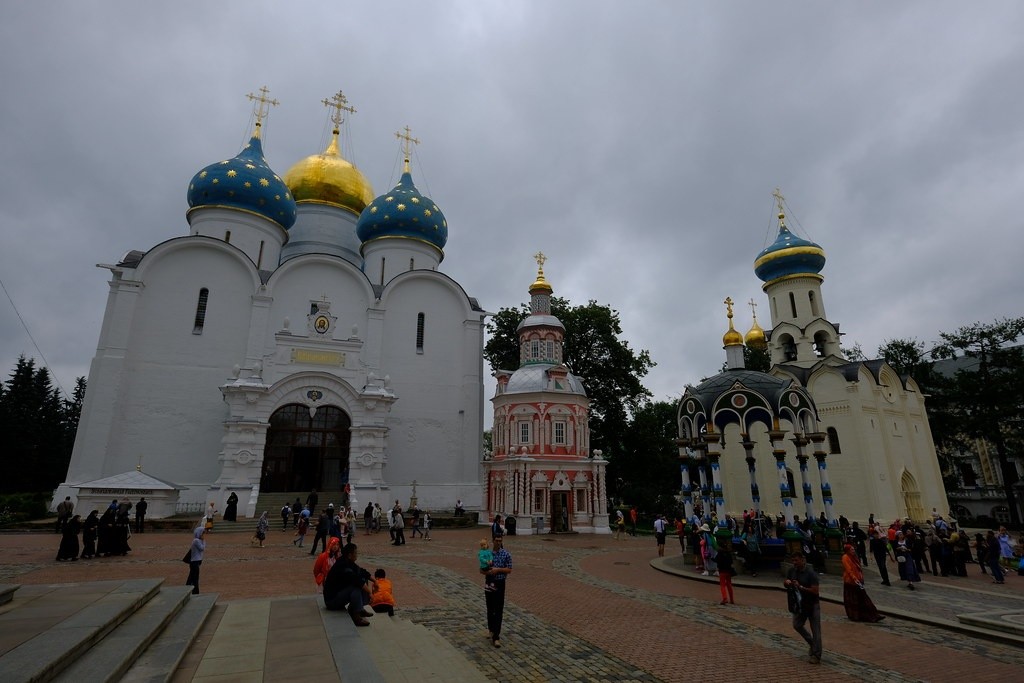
xmin=407 ymin=518 xmax=420 ymax=526
xmin=204 ymin=517 xmax=213 ymax=529
xmin=787 ymin=584 xmax=805 ymax=612
xmin=64 ymin=511 xmax=73 ymax=517
xmin=613 ymin=517 xmax=625 ymax=528
xmin=703 ymin=550 xmax=711 ymax=558
xmin=662 ymin=529 xmax=666 ymax=537
xmin=896 ymin=556 xmax=906 ymax=563
xmin=427 ymin=519 xmax=433 ymax=530
xmin=249 ymin=530 xmax=265 ymax=547
xmin=731 ymin=567 xmax=738 ymax=577
xmin=182 ymin=549 xmax=202 ymax=566
xmin=371 ymin=518 xmax=376 ymax=528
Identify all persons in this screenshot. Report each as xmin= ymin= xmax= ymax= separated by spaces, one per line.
xmin=109 ymin=496 xmax=147 ymax=534
xmin=868 ymin=508 xmax=1024 ymax=591
xmin=562 ymin=507 xmax=568 ymax=530
xmin=614 ymin=511 xmax=628 ymax=540
xmin=56 ymin=510 xmax=132 ymax=562
xmin=652 ymin=515 xmax=670 ymax=557
xmin=370 ymin=568 xmax=397 ymax=617
xmin=55 ymin=496 xmax=74 ymax=533
xmin=479 ymin=514 xmax=513 ymax=647
xmin=323 ymin=543 xmax=379 ymax=626
xmin=629 ymin=507 xmax=637 ymax=536
xmin=783 ymin=552 xmax=822 ymax=664
xmin=364 ymin=499 xmax=431 ymax=545
xmin=454 ymin=499 xmax=466 ymax=517
xmin=313 ymin=537 xmax=341 ymax=593
xmin=186 ymin=527 xmax=206 ymax=594
xmin=207 ymin=492 xmax=238 ymax=533
xmin=253 ymin=488 xmax=357 ymax=556
xmin=675 ymin=509 xmax=886 ymax=623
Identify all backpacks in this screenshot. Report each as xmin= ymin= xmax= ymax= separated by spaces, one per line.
xmin=281 ymin=506 xmax=290 ymax=518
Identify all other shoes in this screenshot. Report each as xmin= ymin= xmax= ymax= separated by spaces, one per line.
xmin=696 ymin=566 xmax=734 ymax=605
xmin=360 ymin=612 xmax=374 ymax=617
xmin=484 ymin=585 xmax=497 ymax=592
xmin=354 ymin=619 xmax=370 ymax=626
xmin=808 ymin=647 xmax=820 ymax=664
xmin=487 ymin=631 xmax=501 ymax=648
xmin=390 ymin=532 xmax=431 ymax=547
xmin=55 ymin=549 xmax=129 ymax=562
xmin=862 ymin=563 xmax=1016 ymax=623
xmin=281 ymin=525 xmax=326 ymax=556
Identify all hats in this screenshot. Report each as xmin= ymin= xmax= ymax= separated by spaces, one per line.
xmin=710 ymin=511 xmax=718 ymax=517
xmin=700 ymin=524 xmax=711 ymax=531
